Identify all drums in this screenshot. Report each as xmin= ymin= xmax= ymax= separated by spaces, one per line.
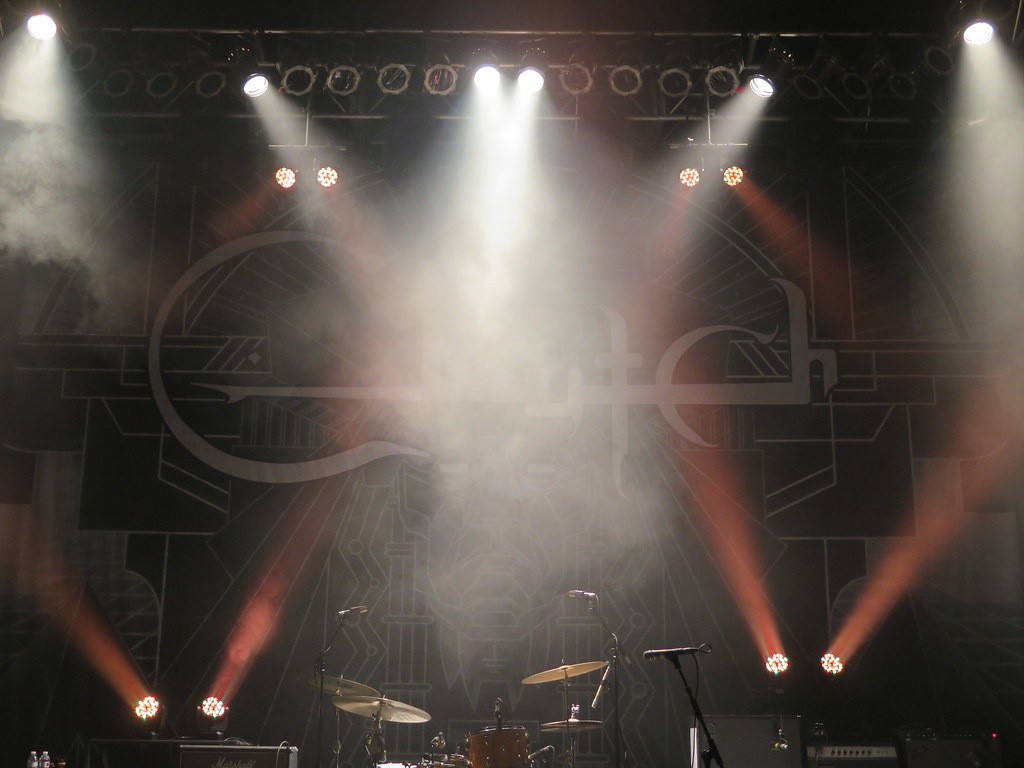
xmin=464 ymin=724 xmax=532 ymax=768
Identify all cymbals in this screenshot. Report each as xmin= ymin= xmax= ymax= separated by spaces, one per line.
xmin=540 ymin=718 xmax=604 ymax=733
xmin=331 ymin=694 xmax=431 ymax=723
xmin=308 ymin=674 xmax=381 ymax=698
xmin=521 ymin=658 xmax=609 ymax=684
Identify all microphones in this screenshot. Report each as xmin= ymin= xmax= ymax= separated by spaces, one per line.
xmin=437 ymin=732 xmax=445 ymax=749
xmin=338 ymin=606 xmax=368 ymax=616
xmin=569 ymin=590 xmax=597 ymax=598
xmin=775 ymin=743 xmax=790 ymax=750
xmin=494 ymin=700 xmax=502 ymax=732
xmin=528 ymin=745 xmax=552 ymax=760
xmin=592 ymin=657 xmax=616 ymax=708
xmin=378 ymin=736 xmax=386 ymax=763
xmin=642 ymin=647 xmax=699 ymax=660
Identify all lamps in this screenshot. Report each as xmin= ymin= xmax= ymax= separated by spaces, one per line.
xmin=65 ymin=0 xmax=1017 ymax=102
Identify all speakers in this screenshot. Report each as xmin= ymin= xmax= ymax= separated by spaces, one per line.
xmin=898 ymin=728 xmax=992 ymax=768
xmin=696 ymin=714 xmax=803 ymax=768
